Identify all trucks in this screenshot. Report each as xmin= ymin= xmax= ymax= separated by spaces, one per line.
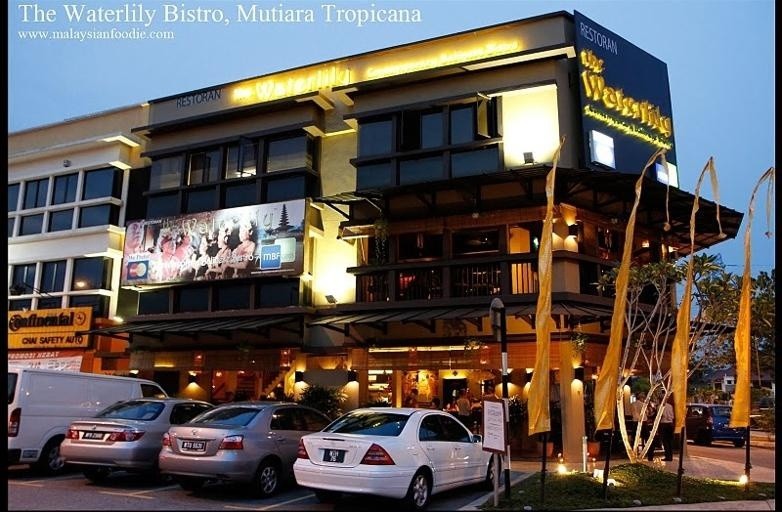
xmin=7 ymin=363 xmax=170 ymax=481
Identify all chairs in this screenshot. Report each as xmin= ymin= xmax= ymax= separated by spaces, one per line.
xmin=471 ymin=406 xmax=483 ymax=435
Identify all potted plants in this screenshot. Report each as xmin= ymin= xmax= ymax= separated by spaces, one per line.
xmin=582 ymin=380 xmax=601 ymax=458
xmin=534 ymin=400 xmax=559 ymax=457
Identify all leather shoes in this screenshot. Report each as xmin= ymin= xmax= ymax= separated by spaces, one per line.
xmin=648 ymin=457 xmax=672 ymax=460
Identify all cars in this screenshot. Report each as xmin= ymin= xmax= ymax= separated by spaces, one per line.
xmin=682 ymin=401 xmax=747 ymax=447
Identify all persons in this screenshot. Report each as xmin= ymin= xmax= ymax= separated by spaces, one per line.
xmin=405 ymin=387 xmax=675 ymax=463
xmin=123 ymin=220 xmax=257 ymax=285
